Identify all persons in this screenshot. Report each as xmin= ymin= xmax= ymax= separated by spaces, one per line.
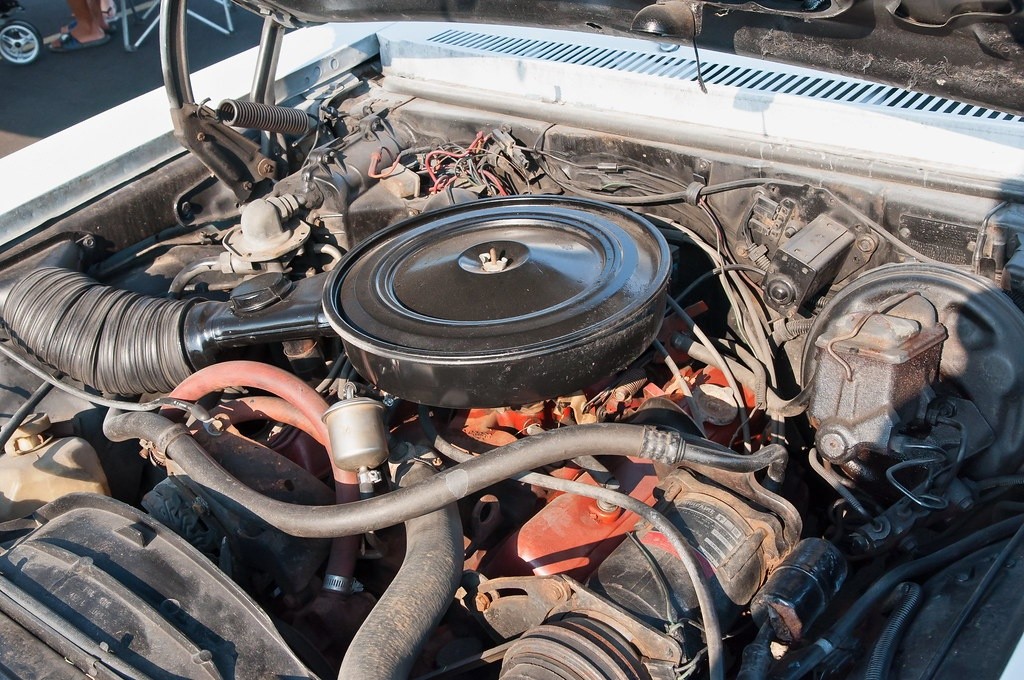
xmin=50 ymin=0 xmax=111 ymax=52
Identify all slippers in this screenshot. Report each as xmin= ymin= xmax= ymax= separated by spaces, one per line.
xmin=48 ymin=31 xmax=111 ymax=53
xmin=64 ymin=19 xmax=118 ymax=35
